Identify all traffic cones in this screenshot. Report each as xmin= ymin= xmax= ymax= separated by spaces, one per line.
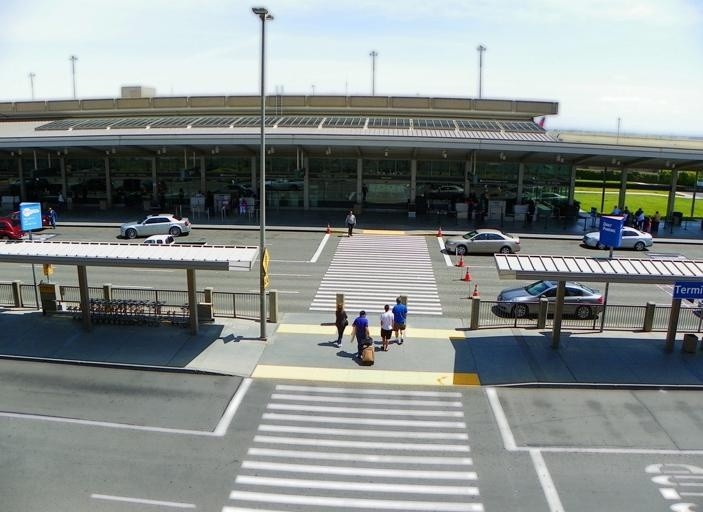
xmin=435 ymin=225 xmax=443 ymax=237
xmin=324 ymin=223 xmax=332 ymax=234
xmin=454 ymin=252 xmax=464 ymax=267
xmin=468 ymin=285 xmax=479 ymax=299
xmin=460 ymin=266 xmax=471 ymax=281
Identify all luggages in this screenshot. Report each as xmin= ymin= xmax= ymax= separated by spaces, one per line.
xmin=359 ymin=345 xmax=374 ymax=366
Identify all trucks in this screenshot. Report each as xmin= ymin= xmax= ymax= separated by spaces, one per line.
xmin=142 ymin=235 xmax=207 ymax=246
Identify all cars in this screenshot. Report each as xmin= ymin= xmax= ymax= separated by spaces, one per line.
xmin=119 ymin=213 xmax=191 ymax=239
xmin=420 ymin=185 xmax=568 ymax=202
xmin=264 ymin=177 xmax=303 ymax=192
xmin=444 ymin=229 xmax=520 ymax=254
xmin=582 ymin=226 xmax=653 ymax=251
xmin=495 ymin=280 xmax=604 ymax=320
xmin=0 ymin=210 xmax=51 ymax=240
xmin=70 ymin=179 xmax=113 ymax=193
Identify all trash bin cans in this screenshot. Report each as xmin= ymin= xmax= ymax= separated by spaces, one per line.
xmin=673 ymin=212 xmax=683 ymax=225
xmin=100 ymin=200 xmax=107 ymax=211
xmin=353 ymin=204 xmax=362 ymax=215
xmin=143 ymin=198 xmax=152 ymax=212
xmin=67 ymin=197 xmax=74 ymax=211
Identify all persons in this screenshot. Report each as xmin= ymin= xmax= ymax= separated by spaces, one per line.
xmin=391 ymin=297 xmax=407 ymax=346
xmin=464 ymin=192 xmax=488 ymax=225
xmin=610 ymin=205 xmax=660 ymax=237
xmin=334 ymin=303 xmax=347 ymax=348
xmin=379 ymin=304 xmax=394 ymax=351
xmin=344 ymin=209 xmax=357 ymax=238
xmin=361 ymin=184 xmax=368 ymax=200
xmin=45 ymin=208 xmax=58 ymax=230
xmin=525 ymin=201 xmax=535 ymax=224
xmin=349 ymin=310 xmax=370 ymax=359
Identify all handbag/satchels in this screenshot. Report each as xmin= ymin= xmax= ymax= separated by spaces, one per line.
xmin=341 ymin=320 xmax=348 ymax=327
xmin=358 ymin=337 xmax=373 ymax=350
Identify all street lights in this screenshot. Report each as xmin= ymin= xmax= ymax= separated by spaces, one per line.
xmin=477 ymin=46 xmax=486 ymax=99
xmin=29 ymin=73 xmax=35 ymax=101
xmin=369 ymin=51 xmax=378 ymax=95
xmin=70 ymin=56 xmax=78 ymax=100
xmin=250 ymin=7 xmax=273 ymax=341
